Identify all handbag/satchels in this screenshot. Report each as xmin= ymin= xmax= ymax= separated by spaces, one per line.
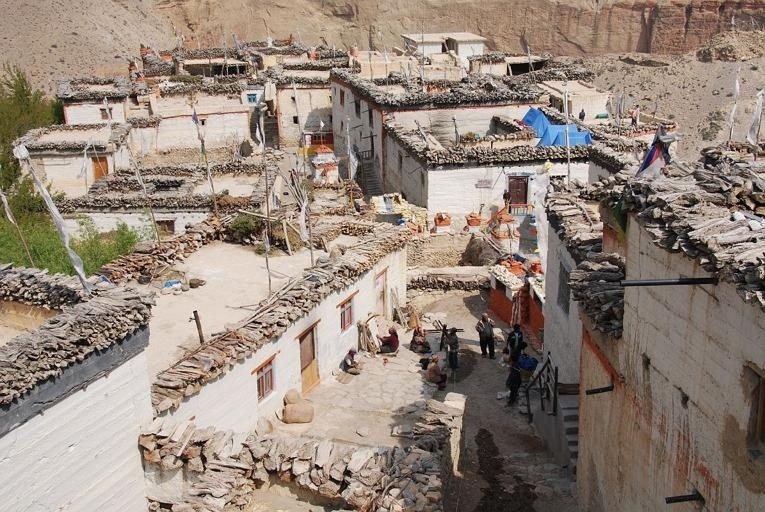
xmin=518 ymin=353 xmax=538 ymax=371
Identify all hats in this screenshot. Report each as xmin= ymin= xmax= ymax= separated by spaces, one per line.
xmin=350 ymin=348 xmax=357 ymax=354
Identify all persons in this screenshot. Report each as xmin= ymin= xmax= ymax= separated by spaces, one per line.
xmin=426 ymin=354 xmax=447 ymax=390
xmin=631 ymin=110 xmax=636 ymax=126
xmin=475 ymin=312 xmax=497 ymax=360
xmin=578 ymin=108 xmax=586 ymax=123
xmin=635 ymin=104 xmax=641 ymax=124
xmin=441 ymin=324 xmax=459 ymax=370
xmin=376 ymin=327 xmax=399 ymax=354
xmin=343 ymin=348 xmax=362 ymax=376
xmin=506 ymin=323 xmax=523 ymax=363
xmin=502 ymin=188 xmax=512 ymax=214
xmin=409 ymin=326 xmax=432 ymax=354
xmin=505 ymin=349 xmax=527 ymax=406
